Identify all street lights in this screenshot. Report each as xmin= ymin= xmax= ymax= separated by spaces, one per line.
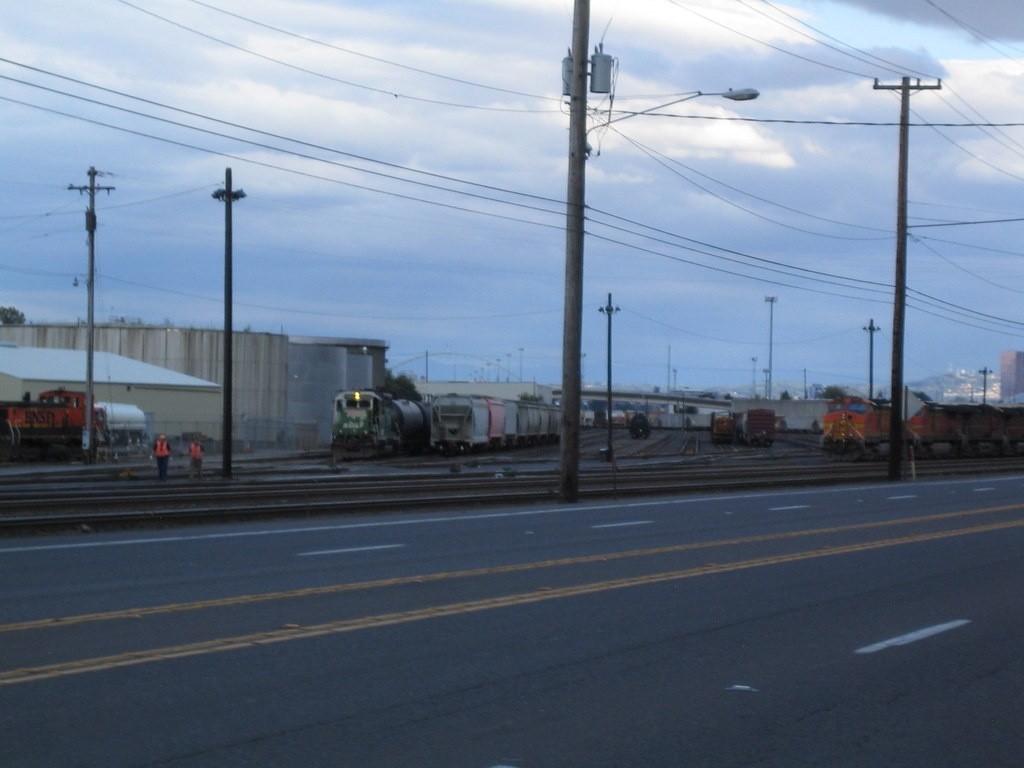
xmin=752 ymin=356 xmax=757 ymax=398
xmin=598 ymin=292 xmax=621 ymax=461
xmin=861 ymin=317 xmax=882 ymax=402
xmin=211 ymin=166 xmax=248 ymax=476
xmin=518 ymin=348 xmax=525 ymax=383
xmin=762 ymin=293 xmax=778 ymax=399
xmin=506 ymin=353 xmax=512 ymax=383
xmin=978 ymin=366 xmax=994 ymax=407
xmin=495 ymin=358 xmax=501 ymax=383
xmin=672 ymin=368 xmax=677 ymax=392
xmin=556 ymin=87 xmax=761 ymax=502
xmin=486 ymin=362 xmax=491 ymax=382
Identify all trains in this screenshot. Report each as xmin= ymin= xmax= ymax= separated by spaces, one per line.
xmin=823 ymin=395 xmax=891 ymax=455
xmin=329 ymin=387 xmax=562 ymax=463
xmin=907 ymin=401 xmax=1024 ymax=458
xmin=710 ymin=407 xmax=776 ymax=447
xmin=0 ymin=387 xmax=110 ymax=462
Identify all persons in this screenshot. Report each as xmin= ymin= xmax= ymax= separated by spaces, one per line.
xmin=185 ymin=436 xmax=207 ymax=482
xmin=150 ymin=434 xmax=172 ymax=481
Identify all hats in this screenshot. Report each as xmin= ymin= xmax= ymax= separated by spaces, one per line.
xmin=160 ymin=434 xmax=165 ymax=440
